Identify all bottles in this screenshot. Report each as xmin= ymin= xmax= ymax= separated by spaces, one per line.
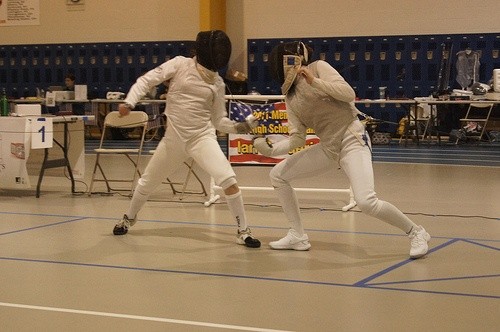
xmin=0 ymin=88 xmax=8 ymax=115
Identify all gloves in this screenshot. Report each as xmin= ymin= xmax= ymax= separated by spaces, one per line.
xmin=254 ymin=138 xmax=278 ymax=158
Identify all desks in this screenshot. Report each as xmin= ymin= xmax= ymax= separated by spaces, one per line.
xmin=0 ymin=115 xmax=85 ymax=198
xmin=8 ymin=94 xmax=500 ymax=147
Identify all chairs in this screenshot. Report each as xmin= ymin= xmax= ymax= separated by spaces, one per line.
xmin=148 ymin=149 xmax=207 ymax=200
xmin=87 ymin=111 xmax=149 ymax=196
xmin=454 ymin=102 xmax=495 ymax=146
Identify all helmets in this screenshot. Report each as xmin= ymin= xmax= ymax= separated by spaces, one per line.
xmin=269 ymin=40 xmax=313 ymax=94
xmin=195 ymin=31 xmax=232 ymax=71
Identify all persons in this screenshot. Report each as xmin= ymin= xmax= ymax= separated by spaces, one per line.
xmin=137 ymin=72 xmax=170 ymax=134
xmin=62 ymin=75 xmax=75 ymax=91
xmin=113 ymin=31 xmax=260 ymax=247
xmin=254 ymin=41 xmax=432 ymax=258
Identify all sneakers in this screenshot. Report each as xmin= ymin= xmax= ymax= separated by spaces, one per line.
xmin=113 ymin=214 xmax=138 ymax=235
xmin=235 ymin=228 xmax=261 ymax=248
xmin=269 ymin=229 xmax=311 ymax=250
xmin=409 ymin=225 xmax=431 ymax=258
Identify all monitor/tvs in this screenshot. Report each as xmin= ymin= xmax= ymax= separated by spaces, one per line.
xmin=49 ymin=86 xmax=63 ymax=92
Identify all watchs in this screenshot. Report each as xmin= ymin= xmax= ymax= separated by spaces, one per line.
xmin=125 ymin=102 xmax=133 ymax=111
